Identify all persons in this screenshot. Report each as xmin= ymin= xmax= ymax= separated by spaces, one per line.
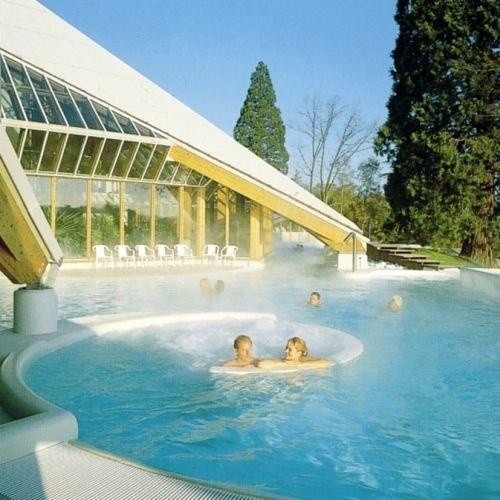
xmin=221 ymin=333 xmax=259 ymax=366
xmin=257 ymin=337 xmax=332 ymax=368
xmin=382 ymin=295 xmax=403 ymax=314
xmin=297 ymin=245 xmax=304 ymax=253
xmin=303 ymin=290 xmax=325 ymax=308
xmin=199 ymin=278 xmax=212 ymax=294
xmin=293 ymin=243 xmax=300 ymax=250
xmin=207 ymin=280 xmax=227 ymax=298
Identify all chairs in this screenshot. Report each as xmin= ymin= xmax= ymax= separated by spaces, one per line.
xmin=92 ymin=244 xmax=115 ymax=270
xmin=220 ymin=245 xmax=239 ymax=264
xmin=135 ymin=244 xmax=157 ymax=268
xmin=154 ymin=244 xmax=175 ymax=267
xmin=202 ymin=245 xmax=220 ymax=264
xmin=174 ymin=244 xmax=194 ymax=268
xmin=116 ymin=245 xmax=135 ymax=269
